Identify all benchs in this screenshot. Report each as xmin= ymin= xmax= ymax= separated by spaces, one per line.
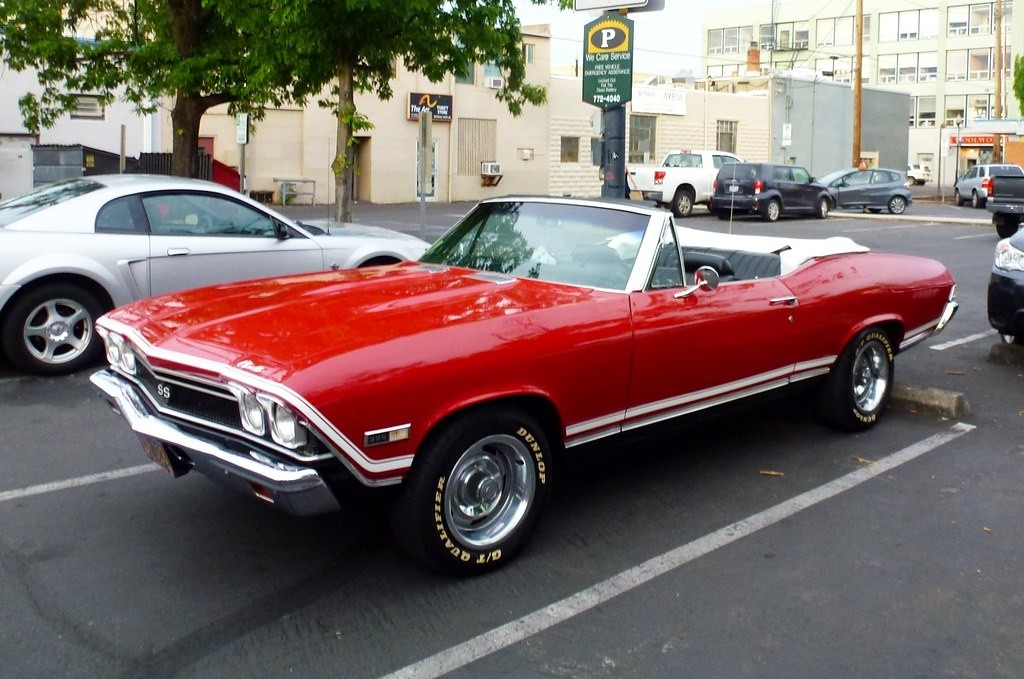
xmin=659 ymin=243 xmax=781 ymax=278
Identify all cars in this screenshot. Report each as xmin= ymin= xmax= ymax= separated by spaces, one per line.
xmin=88 ymin=192 xmax=961 ymax=577
xmin=708 ymin=161 xmax=833 ymax=222
xmin=816 ymin=166 xmax=913 ymax=214
xmin=988 ymin=225 xmax=1023 ymax=344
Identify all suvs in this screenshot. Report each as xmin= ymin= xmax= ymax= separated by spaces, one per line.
xmin=955 ymin=163 xmax=1024 ymax=209
xmin=908 ymin=163 xmax=933 ymax=187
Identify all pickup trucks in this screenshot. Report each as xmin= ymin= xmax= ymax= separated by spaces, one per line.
xmin=984 ymin=174 xmax=1024 ymax=237
xmin=628 ymin=149 xmax=757 ymax=217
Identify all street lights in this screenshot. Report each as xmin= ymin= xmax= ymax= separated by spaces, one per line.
xmin=955 ymin=113 xmax=962 ymax=186
xmin=937 ymin=123 xmax=947 ymax=204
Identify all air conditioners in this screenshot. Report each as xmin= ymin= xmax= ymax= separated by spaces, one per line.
xmin=482 ymin=163 xmax=501 ymax=175
xmin=484 ymin=77 xmax=503 ymax=88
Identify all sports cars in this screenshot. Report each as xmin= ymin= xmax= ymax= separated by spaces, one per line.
xmin=0 ymin=171 xmax=453 ymax=376
